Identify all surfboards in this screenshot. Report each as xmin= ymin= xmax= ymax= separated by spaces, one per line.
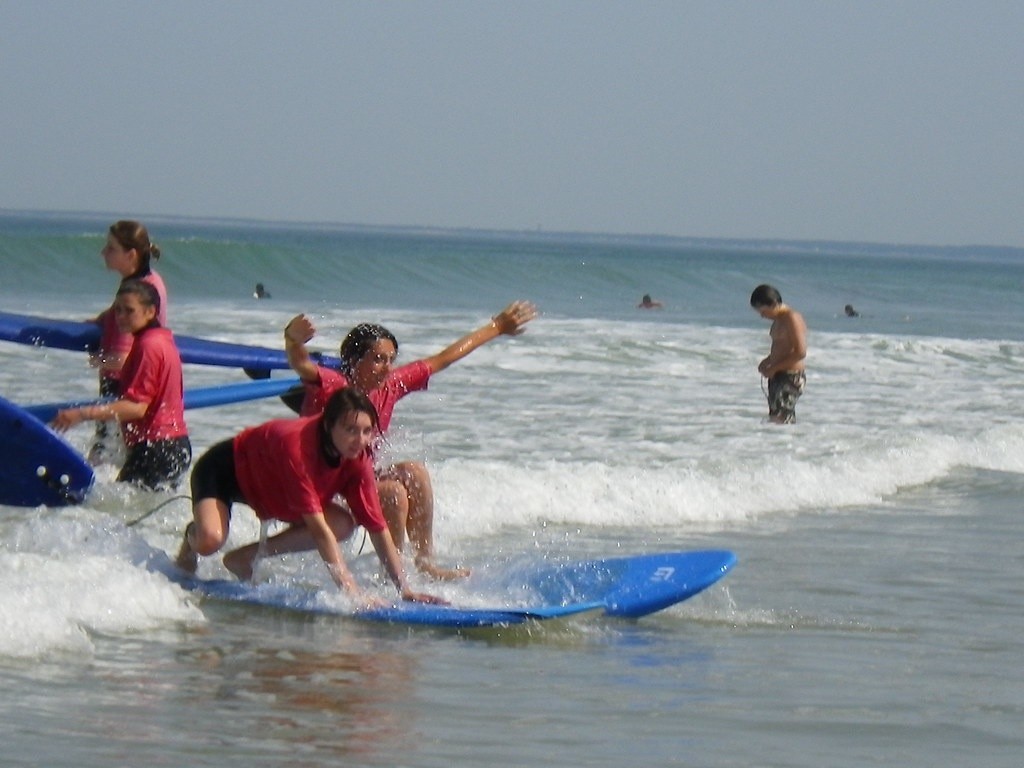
xmin=160 ymin=568 xmax=610 ymax=627
xmin=0 ymin=394 xmax=98 ymax=509
xmin=437 ymin=546 xmax=741 ymax=621
xmin=22 ymin=379 xmax=307 ymax=426
xmin=0 ymin=310 xmax=348 ymax=373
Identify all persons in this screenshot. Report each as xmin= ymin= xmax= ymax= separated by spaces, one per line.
xmin=285 ymin=299 xmax=538 ymax=585
xmin=252 ymin=283 xmax=270 ymax=298
xmin=83 ymin=220 xmax=167 ymax=466
xmin=844 ymin=305 xmax=861 ymax=318
xmin=639 ymin=295 xmax=662 ymax=308
xmin=52 ymin=278 xmax=192 ymax=493
xmin=176 ymin=389 xmax=450 ymax=612
xmin=749 ymin=284 xmax=806 ymax=424
